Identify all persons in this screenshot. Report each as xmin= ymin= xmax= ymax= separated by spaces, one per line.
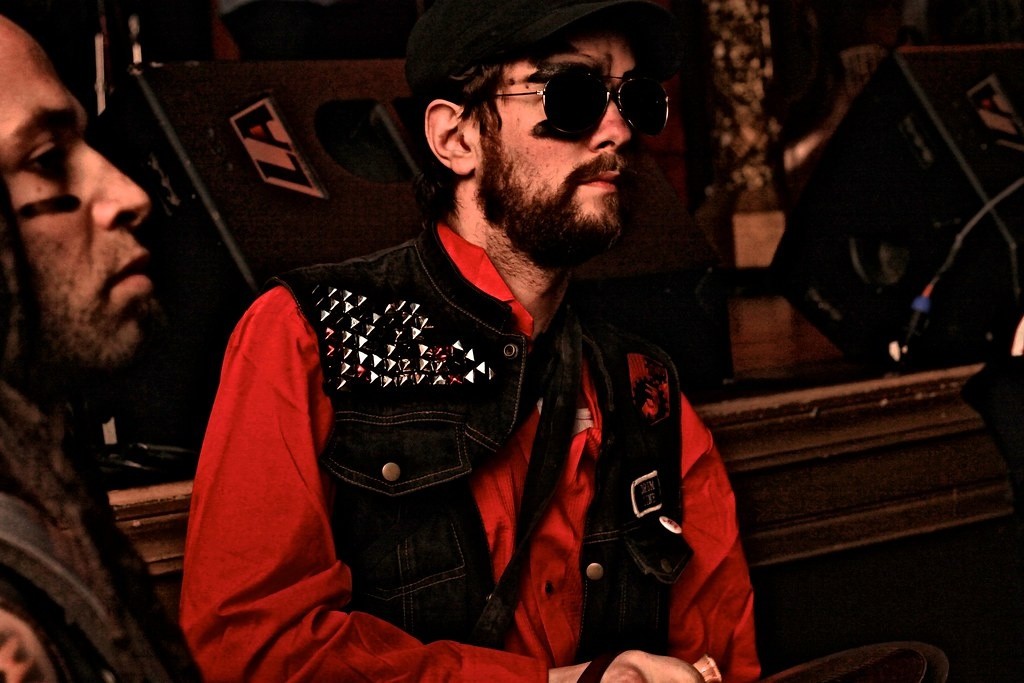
xmin=770 ymin=0 xmax=931 ymax=207
xmin=176 ymin=0 xmax=763 ymax=683
xmin=0 ymin=11 xmax=206 ymax=683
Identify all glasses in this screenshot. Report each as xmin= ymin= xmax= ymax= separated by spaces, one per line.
xmin=495 ymin=65 xmax=670 ymax=140
